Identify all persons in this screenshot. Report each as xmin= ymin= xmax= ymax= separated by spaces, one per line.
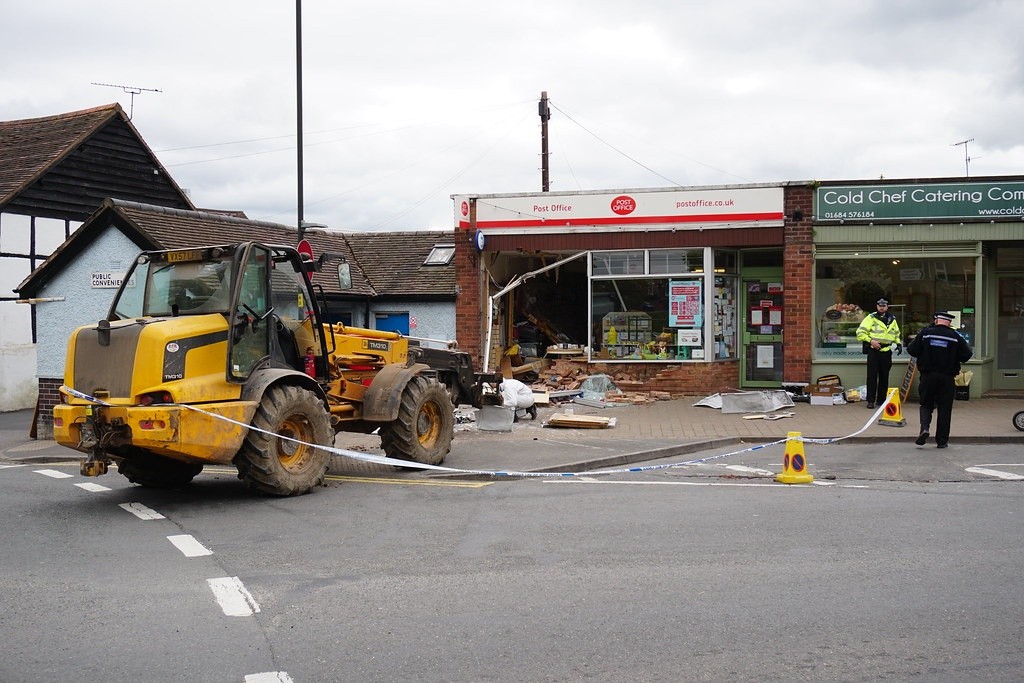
xmin=856 ymin=298 xmax=902 ymax=409
xmin=906 ymin=311 xmax=973 ymax=448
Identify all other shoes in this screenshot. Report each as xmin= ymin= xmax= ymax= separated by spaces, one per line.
xmin=876 ymin=400 xmax=885 ymax=404
xmin=937 ymin=440 xmax=948 ymax=448
xmin=529 ymin=405 xmax=537 ymax=420
xmin=513 ymin=414 xmax=519 ymax=423
xmin=867 ymin=401 xmax=874 ymax=408
xmin=916 ymin=427 xmax=929 ymax=445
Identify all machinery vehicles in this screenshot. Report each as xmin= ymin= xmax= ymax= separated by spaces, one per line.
xmin=52 ymin=241 xmax=504 ymax=498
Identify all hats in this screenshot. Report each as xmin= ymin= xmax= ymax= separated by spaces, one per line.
xmin=933 ymin=312 xmax=955 ymax=323
xmin=874 ymin=297 xmax=889 ymax=305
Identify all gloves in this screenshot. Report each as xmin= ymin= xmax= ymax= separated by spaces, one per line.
xmin=895 ymin=344 xmax=903 ymax=356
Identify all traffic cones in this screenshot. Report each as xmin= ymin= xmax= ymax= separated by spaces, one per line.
xmin=776 ymin=431 xmax=814 ymax=484
xmin=878 ymin=388 xmax=906 ymax=427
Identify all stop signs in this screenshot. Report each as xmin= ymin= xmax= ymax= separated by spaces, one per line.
xmin=298 ymin=240 xmax=315 ymax=280
